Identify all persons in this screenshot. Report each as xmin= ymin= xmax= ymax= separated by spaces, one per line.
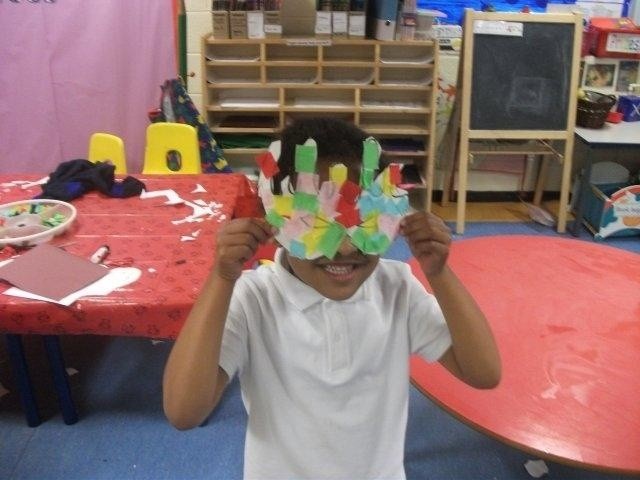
xmin=162 ymin=111 xmax=505 ymax=479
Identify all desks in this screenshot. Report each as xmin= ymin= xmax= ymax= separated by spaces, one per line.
xmin=0 ymin=172 xmax=247 ymax=421
xmin=401 ymin=235 xmax=640 ymax=478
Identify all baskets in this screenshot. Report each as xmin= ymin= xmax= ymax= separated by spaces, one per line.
xmin=576 ymin=90 xmax=616 ymax=129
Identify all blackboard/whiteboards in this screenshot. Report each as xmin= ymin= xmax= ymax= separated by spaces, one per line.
xmin=468 ymin=22 xmax=578 ymax=131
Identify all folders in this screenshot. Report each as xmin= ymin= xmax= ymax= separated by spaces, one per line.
xmin=373 ymin=1 xmax=398 ymax=41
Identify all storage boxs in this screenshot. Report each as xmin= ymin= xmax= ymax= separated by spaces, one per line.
xmin=569 ymin=144 xmax=639 ymax=241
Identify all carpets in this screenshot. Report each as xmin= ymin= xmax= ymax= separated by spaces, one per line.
xmin=0 ymin=218 xmax=640 ymax=480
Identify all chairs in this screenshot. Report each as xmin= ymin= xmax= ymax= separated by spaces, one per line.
xmin=141 ymin=120 xmax=205 ymax=176
xmin=86 ymin=131 xmax=127 ymax=174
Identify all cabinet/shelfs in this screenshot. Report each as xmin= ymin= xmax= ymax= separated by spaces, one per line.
xmin=202 ymin=34 xmax=440 ymax=219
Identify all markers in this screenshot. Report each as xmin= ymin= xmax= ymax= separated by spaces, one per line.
xmin=88 ymin=244 xmax=112 ymax=265
